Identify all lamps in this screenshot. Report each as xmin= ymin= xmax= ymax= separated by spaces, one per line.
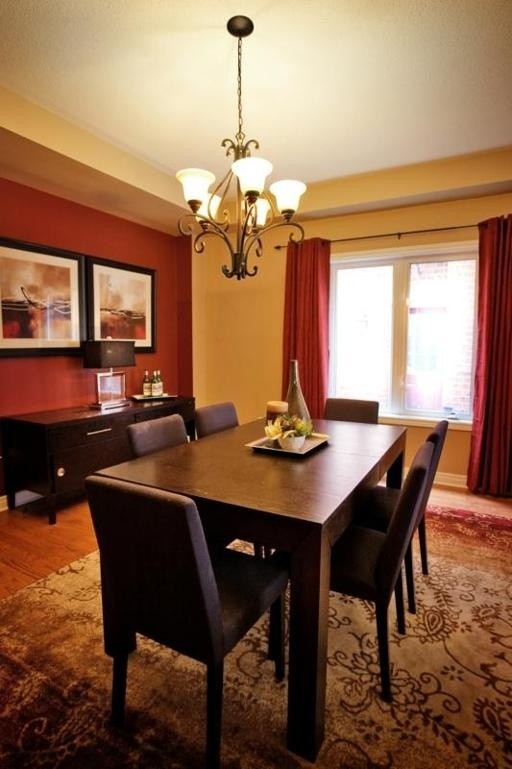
xmin=83 ymin=339 xmax=137 ymax=414
xmin=177 ymin=14 xmax=304 ymax=282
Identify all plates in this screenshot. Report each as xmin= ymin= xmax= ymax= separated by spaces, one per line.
xmin=243 ymin=432 xmax=331 ymax=454
xmin=131 ymin=393 xmax=178 ymax=400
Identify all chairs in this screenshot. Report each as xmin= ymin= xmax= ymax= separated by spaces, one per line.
xmin=86 ymin=475 xmax=290 ymax=769
xmin=265 ymin=443 xmax=436 ymax=700
xmin=127 ymin=414 xmax=189 ymax=458
xmin=362 ymin=420 xmax=450 ymax=616
xmin=325 ymin=398 xmax=378 ymax=423
xmin=195 ymin=402 xmax=238 ymax=438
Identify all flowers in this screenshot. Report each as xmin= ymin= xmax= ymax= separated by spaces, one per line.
xmin=265 ymin=415 xmax=313 ymax=438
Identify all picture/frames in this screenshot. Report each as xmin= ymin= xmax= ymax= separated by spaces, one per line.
xmin=83 ymin=254 xmax=156 ymax=355
xmin=0 ymin=236 xmax=87 ymax=357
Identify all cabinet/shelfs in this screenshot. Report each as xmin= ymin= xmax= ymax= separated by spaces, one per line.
xmin=0 ymin=398 xmax=196 ymax=525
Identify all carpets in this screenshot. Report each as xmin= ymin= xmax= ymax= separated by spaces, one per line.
xmin=0 ymin=507 xmax=512 ymax=769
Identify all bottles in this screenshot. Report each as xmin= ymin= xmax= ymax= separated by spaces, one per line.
xmin=286 ymin=360 xmax=312 ymax=429
xmin=142 ymin=370 xmax=152 ymax=397
xmin=156 ymin=369 xmax=163 ymax=394
xmin=265 ymin=401 xmax=288 ymax=435
xmin=152 ymin=371 xmax=159 ymax=397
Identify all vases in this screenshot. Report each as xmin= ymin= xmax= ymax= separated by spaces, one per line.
xmin=279 ymin=436 xmax=305 ymax=448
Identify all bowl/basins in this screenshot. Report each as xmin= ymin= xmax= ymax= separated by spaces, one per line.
xmin=277 ymin=435 xmax=307 ymax=450
